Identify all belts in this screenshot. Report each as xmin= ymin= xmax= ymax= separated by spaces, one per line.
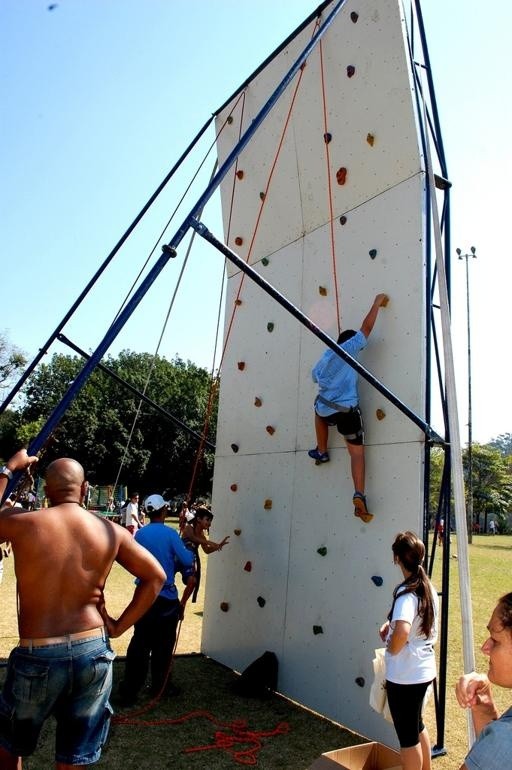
xmin=19 ymin=626 xmax=107 ymax=647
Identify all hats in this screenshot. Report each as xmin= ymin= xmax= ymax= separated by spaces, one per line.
xmin=145 ymin=494 xmax=170 ymax=513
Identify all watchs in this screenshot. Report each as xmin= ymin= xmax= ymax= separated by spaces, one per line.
xmin=2 ymin=466 xmax=13 ymax=479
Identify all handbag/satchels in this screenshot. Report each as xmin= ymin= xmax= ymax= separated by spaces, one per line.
xmin=369 ymin=648 xmax=432 ymax=722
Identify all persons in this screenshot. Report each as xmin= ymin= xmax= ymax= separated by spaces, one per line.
xmin=438 ymin=515 xmax=445 ymax=546
xmin=489 ymin=519 xmax=494 ymax=534
xmin=1 ymin=448 xmax=166 ymax=770
xmin=112 ymin=494 xmax=196 ymax=707
xmin=4 ymin=489 xmax=211 ymax=557
xmin=307 ymin=294 xmax=386 ymax=515
xmin=379 ymin=531 xmax=439 ymax=770
xmin=180 ymin=508 xmax=230 ymax=620
xmin=456 ymin=593 xmax=512 ymax=770
xmin=474 ymin=521 xmax=480 ymax=534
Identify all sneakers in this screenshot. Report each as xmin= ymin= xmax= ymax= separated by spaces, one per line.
xmin=308 ymin=446 xmax=329 ymax=462
xmin=352 ymin=492 xmax=368 ymax=517
